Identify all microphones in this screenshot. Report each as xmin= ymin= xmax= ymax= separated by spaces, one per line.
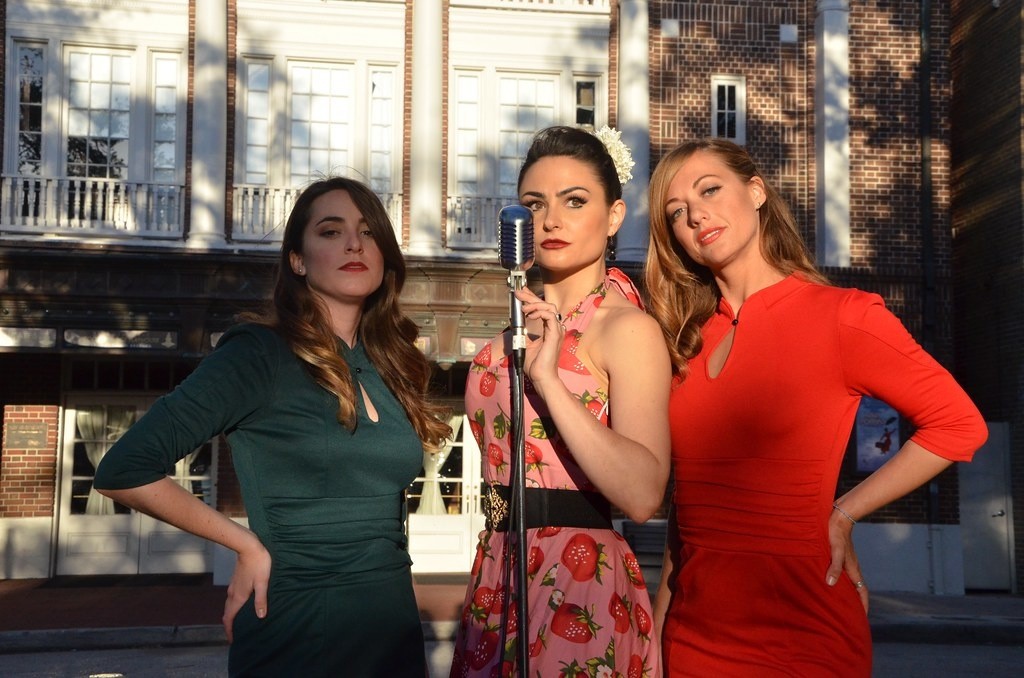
xmin=498 ymin=205 xmax=536 ymax=369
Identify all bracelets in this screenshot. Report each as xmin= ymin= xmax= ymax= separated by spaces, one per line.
xmin=833 ymin=505 xmax=856 ymax=525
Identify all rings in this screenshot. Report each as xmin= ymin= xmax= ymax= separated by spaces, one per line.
xmin=557 ymin=313 xmax=561 ymax=321
xmin=856 ymin=580 xmax=863 ymax=587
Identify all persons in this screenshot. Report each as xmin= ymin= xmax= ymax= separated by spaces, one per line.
xmin=447 ymin=126 xmax=670 ymax=678
xmin=647 ymin=107 xmax=987 ymax=678
xmin=90 ymin=178 xmax=426 ymax=678
